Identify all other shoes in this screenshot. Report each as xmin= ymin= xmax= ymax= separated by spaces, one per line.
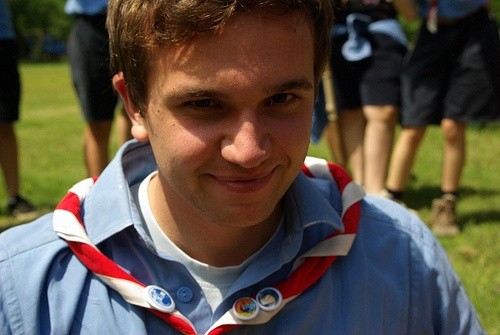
xmin=8 ymin=197 xmax=39 ymax=220
xmin=430 ymin=194 xmax=460 ymax=237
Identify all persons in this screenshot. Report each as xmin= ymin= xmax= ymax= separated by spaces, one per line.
xmin=323 ymin=0 xmax=410 ymax=202
xmin=385 ymin=0 xmax=500 ymax=238
xmin=65 ymin=0 xmax=122 ymax=183
xmin=1 ymin=0 xmax=485 ymax=335
xmin=0 ymin=0 xmax=39 ymax=217
